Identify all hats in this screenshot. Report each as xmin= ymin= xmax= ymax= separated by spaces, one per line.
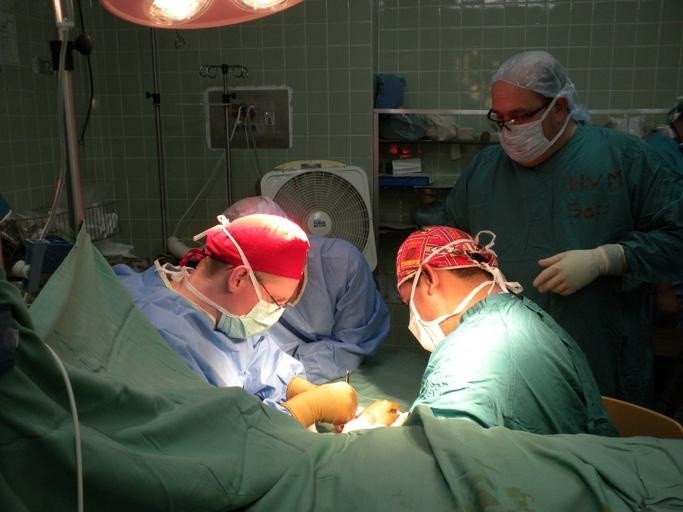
xmin=205 ymin=213 xmax=308 ymax=282
xmin=396 ymin=228 xmax=487 ymax=289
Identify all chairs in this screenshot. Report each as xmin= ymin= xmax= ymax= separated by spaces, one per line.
xmin=601 ymin=395 xmax=683 ymax=441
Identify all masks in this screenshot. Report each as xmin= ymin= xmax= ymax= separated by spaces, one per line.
xmin=408 ymin=266 xmax=443 ymax=352
xmin=218 ymin=265 xmax=285 ymax=340
xmin=497 ymin=97 xmax=557 ymax=166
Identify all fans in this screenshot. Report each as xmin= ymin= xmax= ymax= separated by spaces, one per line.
xmin=259 ymin=160 xmax=378 ymax=275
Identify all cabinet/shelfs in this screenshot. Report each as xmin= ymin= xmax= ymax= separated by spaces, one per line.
xmin=373 ymin=108 xmax=671 ymax=256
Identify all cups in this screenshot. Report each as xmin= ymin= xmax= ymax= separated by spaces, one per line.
xmin=456 ymin=128 xmax=473 ymax=142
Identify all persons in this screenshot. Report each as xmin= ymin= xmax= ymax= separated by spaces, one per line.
xmin=337 ymin=224 xmax=616 ymax=438
xmin=112 ymin=215 xmax=354 ymax=428
xmin=425 ymin=49 xmax=681 ymax=410
xmin=218 ymin=197 xmax=391 ymax=383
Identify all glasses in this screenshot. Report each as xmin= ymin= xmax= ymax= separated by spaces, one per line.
xmin=486 ymin=107 xmax=543 ymax=133
xmin=259 ymin=281 xmax=287 ymax=313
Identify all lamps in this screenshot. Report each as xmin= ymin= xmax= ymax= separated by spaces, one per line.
xmin=102 ymin=0 xmax=303 ymax=29
